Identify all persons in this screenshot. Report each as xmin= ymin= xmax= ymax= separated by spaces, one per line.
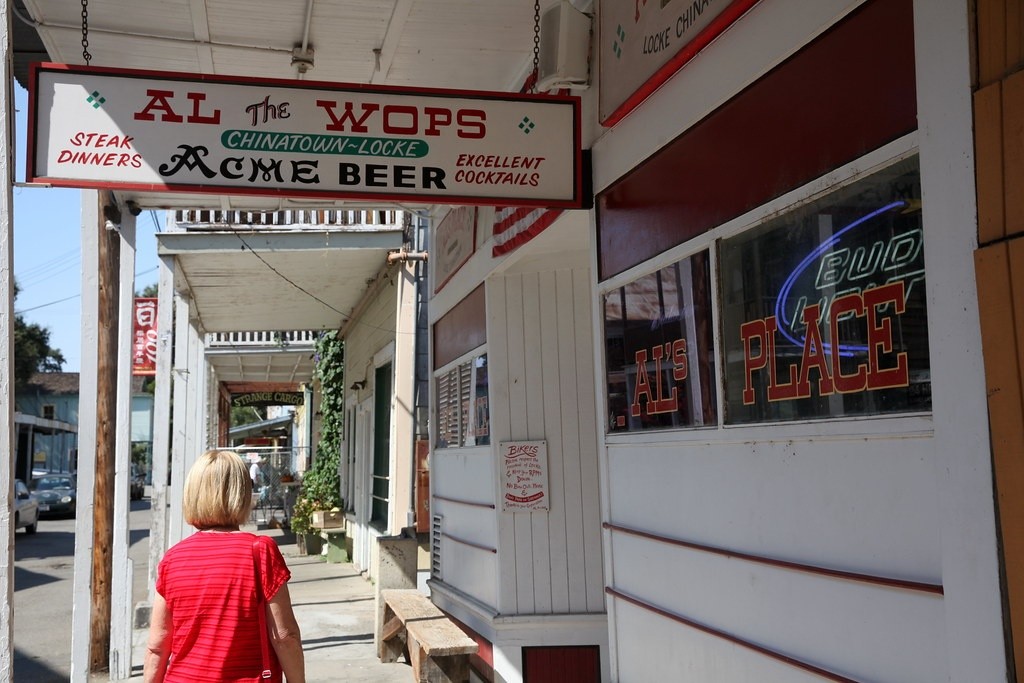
xmin=141 ymin=448 xmax=306 ymax=683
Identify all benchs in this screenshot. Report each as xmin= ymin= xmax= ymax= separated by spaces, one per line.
xmin=379 ymin=589 xmax=480 ymax=683
xmin=309 ymin=525 xmax=349 ymax=564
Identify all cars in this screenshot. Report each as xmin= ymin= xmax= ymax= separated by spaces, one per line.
xmin=129 ymin=463 xmax=147 ymax=502
xmin=14 ymin=479 xmax=39 ymax=537
xmin=29 ymin=473 xmax=77 ymax=519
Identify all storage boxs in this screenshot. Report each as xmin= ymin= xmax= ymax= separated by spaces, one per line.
xmin=313 ymin=510 xmax=343 ymax=528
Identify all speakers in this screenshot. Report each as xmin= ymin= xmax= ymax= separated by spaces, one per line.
xmin=536 ymin=0 xmax=591 ymax=94
